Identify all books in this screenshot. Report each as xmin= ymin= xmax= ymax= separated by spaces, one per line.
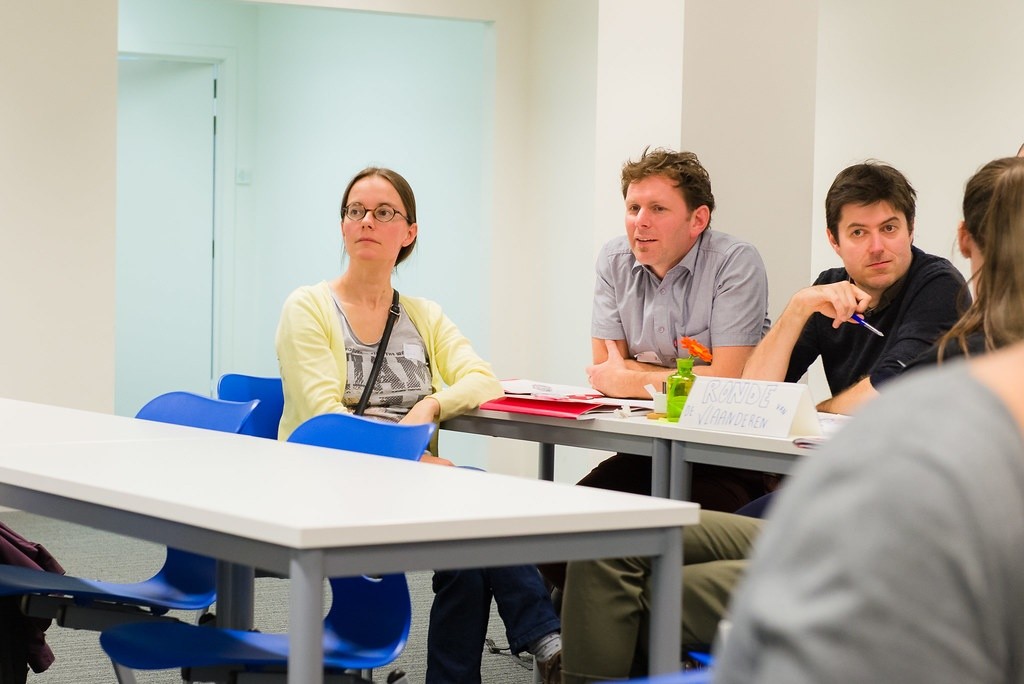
xmin=480 ymin=389 xmax=638 ymax=423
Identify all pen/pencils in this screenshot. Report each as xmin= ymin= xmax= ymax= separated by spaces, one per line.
xmin=663 ymin=381 xmax=666 ymax=394
xmin=852 ymin=314 xmax=884 ymax=337
xmin=505 ymin=392 xmax=531 ymax=395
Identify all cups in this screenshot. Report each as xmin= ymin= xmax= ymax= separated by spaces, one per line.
xmin=652 ymin=392 xmax=667 ymax=413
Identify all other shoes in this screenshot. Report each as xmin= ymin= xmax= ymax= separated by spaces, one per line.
xmin=531 ymin=632 xmax=562 ymax=684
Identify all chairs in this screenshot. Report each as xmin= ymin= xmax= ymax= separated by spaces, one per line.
xmin=0 ymin=391 xmax=262 ymax=684
xmin=219 ymin=375 xmax=284 ymax=442
xmin=99 ymin=413 xmax=436 ymax=684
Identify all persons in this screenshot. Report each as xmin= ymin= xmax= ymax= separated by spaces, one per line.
xmin=743 ymin=165 xmax=976 ymax=414
xmin=276 ymin=167 xmax=563 ymax=684
xmin=546 ymin=145 xmax=791 ymax=601
xmin=563 ymin=157 xmax=1024 ymax=684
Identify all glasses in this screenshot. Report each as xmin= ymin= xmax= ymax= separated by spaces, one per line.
xmin=342 ymin=202 xmax=411 ymax=222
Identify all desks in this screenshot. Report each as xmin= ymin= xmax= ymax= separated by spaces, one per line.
xmin=0 ymin=395 xmax=700 ymax=684
xmin=438 ymin=377 xmax=853 ymax=678
